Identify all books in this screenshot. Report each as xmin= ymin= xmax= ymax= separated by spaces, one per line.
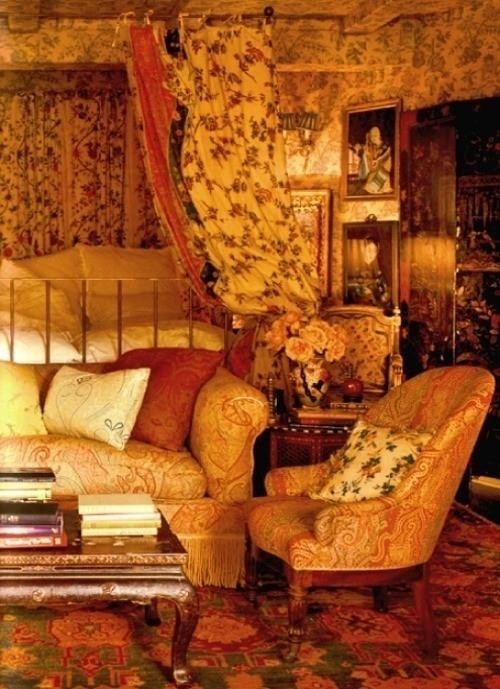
xmin=0 ymin=467 xmax=163 ymax=547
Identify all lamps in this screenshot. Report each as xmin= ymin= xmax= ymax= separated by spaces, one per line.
xmin=276 ymin=108 xmax=324 ymax=155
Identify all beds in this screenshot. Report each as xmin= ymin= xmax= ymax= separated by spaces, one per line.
xmin=2 ymin=242 xmax=321 ymax=364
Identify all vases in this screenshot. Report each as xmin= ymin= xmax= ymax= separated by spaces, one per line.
xmin=293 ymin=351 xmax=333 ymax=405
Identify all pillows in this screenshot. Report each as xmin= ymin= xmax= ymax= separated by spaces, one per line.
xmin=104 ymin=345 xmax=232 ymax=453
xmin=42 ymin=365 xmax=152 ymax=452
xmin=0 ymin=240 xmax=82 ymax=313
xmin=80 ymin=242 xmax=186 ymax=295
xmin=2 ymin=365 xmax=46 ymax=438
xmin=304 ymin=415 xmax=436 ymax=503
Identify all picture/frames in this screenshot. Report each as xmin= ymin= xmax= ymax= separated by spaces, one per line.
xmin=337 ymin=98 xmax=401 ymax=198
xmin=289 ymin=190 xmax=332 ymax=296
xmin=343 ymin=221 xmax=399 ymax=306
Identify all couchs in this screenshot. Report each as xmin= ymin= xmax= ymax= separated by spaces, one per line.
xmin=247 ymin=362 xmax=495 ymax=661
xmin=4 ymin=363 xmax=273 ymax=593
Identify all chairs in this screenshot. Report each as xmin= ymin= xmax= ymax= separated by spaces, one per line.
xmin=279 ymin=305 xmax=402 ymax=401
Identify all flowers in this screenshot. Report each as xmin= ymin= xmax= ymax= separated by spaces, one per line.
xmin=283 ymin=314 xmax=353 ymax=364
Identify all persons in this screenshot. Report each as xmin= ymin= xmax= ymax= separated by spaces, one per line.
xmin=355 ymin=233 xmax=379 ymax=274
xmin=360 ymin=125 xmax=391 ymax=192
